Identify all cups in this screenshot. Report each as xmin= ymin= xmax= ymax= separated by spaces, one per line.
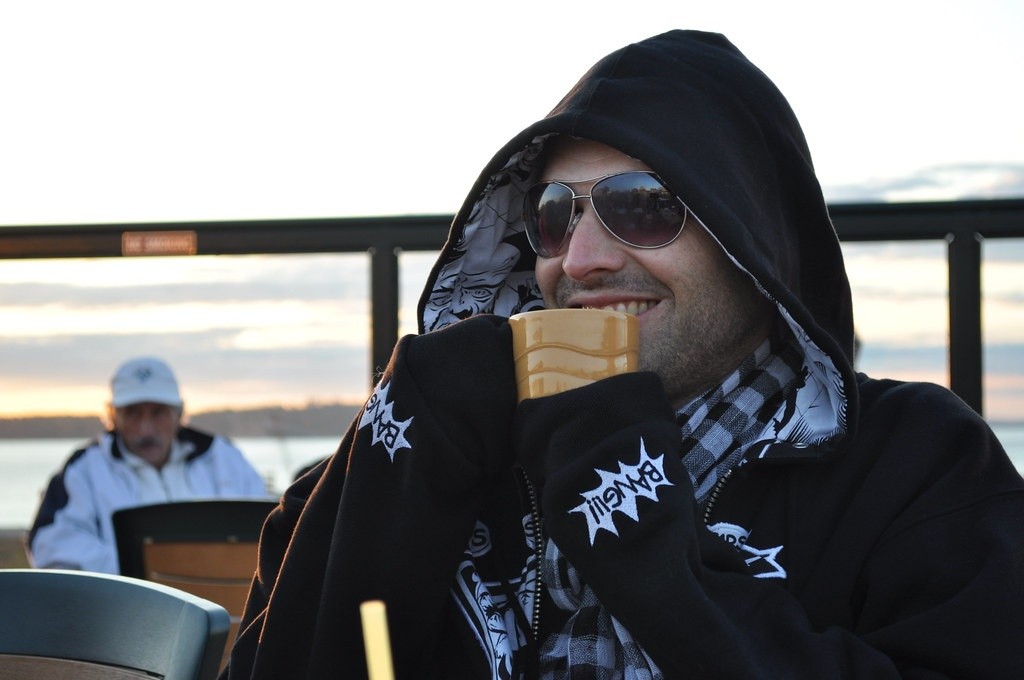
xmin=506 ymin=308 xmax=638 ymax=409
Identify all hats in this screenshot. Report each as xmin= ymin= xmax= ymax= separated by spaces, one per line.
xmin=110 ymin=357 xmax=182 ymax=410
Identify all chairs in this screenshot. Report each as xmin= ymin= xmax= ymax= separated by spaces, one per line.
xmin=0 ymin=567 xmax=230 ymax=680
xmin=108 ymin=499 xmax=283 ymax=679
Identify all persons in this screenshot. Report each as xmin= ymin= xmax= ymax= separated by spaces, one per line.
xmin=214 ymin=27 xmax=1023 ymax=680
xmin=24 ymin=359 xmax=280 ymax=575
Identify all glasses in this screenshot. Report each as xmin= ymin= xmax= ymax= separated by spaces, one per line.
xmin=521 ymin=169 xmax=690 ymax=258
